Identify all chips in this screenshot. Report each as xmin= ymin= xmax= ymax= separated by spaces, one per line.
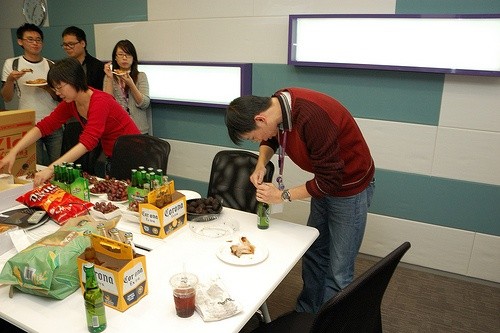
xmin=39 ymin=189 xmax=66 ymax=212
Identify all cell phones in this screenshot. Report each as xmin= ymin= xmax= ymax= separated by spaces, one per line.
xmin=27 ymin=211 xmax=47 ymax=224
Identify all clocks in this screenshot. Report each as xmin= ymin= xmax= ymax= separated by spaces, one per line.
xmin=23 ymin=0 xmax=46 ymax=26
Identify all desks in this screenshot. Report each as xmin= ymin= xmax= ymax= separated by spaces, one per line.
xmin=0 ymin=164 xmax=320 ymax=333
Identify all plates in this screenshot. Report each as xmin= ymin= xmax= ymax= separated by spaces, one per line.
xmin=186 ymin=204 xmax=222 ymax=222
xmin=23 ymin=81 xmax=48 ymax=86
xmin=215 ymin=238 xmax=268 ymax=265
xmin=0 ymin=207 xmax=49 ymax=231
xmin=176 ymin=190 xmax=201 ymax=200
xmin=88 ymin=179 xmax=138 ymax=223
xmin=112 ymin=71 xmax=131 ymax=76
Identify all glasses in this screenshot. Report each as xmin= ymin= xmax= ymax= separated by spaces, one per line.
xmin=116 ymin=53 xmax=132 ymax=58
xmin=51 ymin=84 xmax=66 ymax=92
xmin=23 ymin=38 xmax=42 ymax=44
xmin=61 ymin=41 xmax=78 ymax=48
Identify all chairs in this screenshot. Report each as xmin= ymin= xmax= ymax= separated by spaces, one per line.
xmin=207 ymin=150 xmax=275 ymax=326
xmin=248 ymin=241 xmax=411 ymax=333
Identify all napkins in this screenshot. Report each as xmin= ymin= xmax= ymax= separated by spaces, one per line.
xmin=195 ymin=278 xmax=244 ymax=323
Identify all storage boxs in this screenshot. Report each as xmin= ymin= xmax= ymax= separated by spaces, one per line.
xmin=127 ymin=172 xmax=162 ymax=212
xmin=0 ymin=174 xmax=33 ymax=211
xmin=139 ymin=180 xmax=187 ymax=239
xmin=77 ymin=231 xmax=149 ymax=312
xmin=50 ymin=167 xmax=91 ymax=201
xmin=0 ymin=109 xmax=36 ymax=178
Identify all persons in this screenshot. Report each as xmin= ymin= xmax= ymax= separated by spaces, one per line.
xmin=37 ymin=26 xmax=105 ymax=181
xmin=225 ymin=88 xmax=376 ymax=315
xmin=0 ymin=56 xmax=142 ymax=191
xmin=0 ymin=23 xmax=64 ymax=168
xmin=103 ymin=40 xmax=153 ymax=139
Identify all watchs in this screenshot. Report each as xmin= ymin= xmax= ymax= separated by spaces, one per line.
xmin=281 ymin=188 xmax=292 ymax=203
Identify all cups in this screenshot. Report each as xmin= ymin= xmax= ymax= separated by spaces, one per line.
xmin=170 ymin=273 xmax=198 ymax=318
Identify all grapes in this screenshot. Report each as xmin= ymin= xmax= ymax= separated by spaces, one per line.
xmin=82 ymin=172 xmax=129 ymax=214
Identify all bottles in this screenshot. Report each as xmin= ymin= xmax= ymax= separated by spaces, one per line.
xmin=132 ymin=165 xmax=172 ymax=209
xmin=84 ymin=223 xmax=136 ymax=264
xmin=257 ymin=203 xmax=270 ymax=229
xmin=84 ymin=263 xmax=107 ymax=333
xmin=53 ymin=162 xmax=84 ymax=183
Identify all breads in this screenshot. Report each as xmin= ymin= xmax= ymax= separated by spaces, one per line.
xmin=187 ymin=196 xmax=221 ymax=222
